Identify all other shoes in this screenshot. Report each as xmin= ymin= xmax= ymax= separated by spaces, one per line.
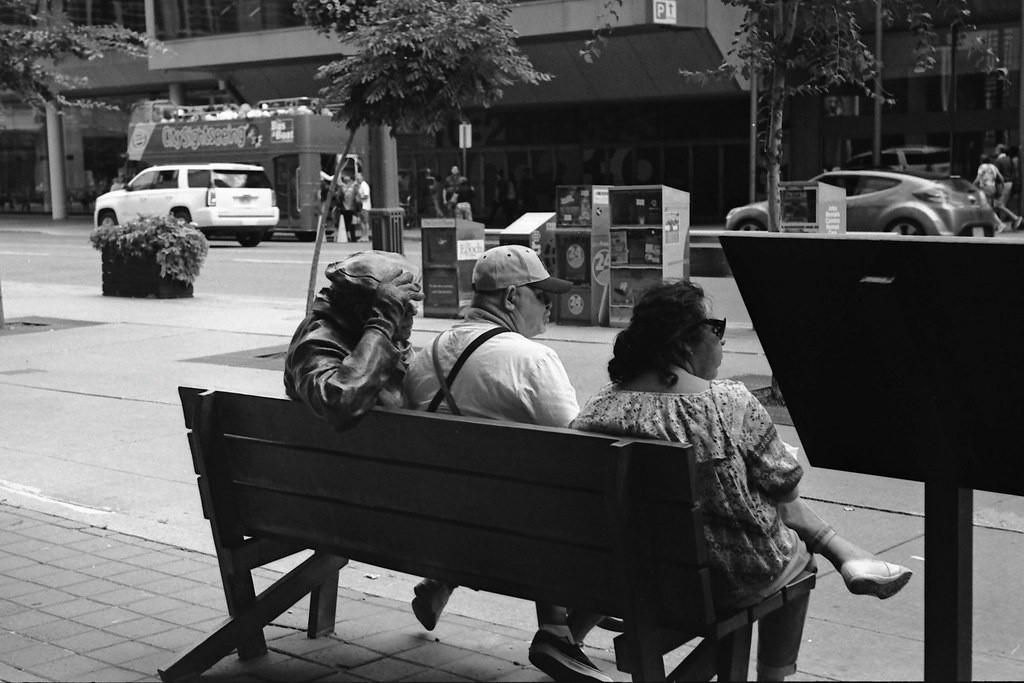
xmin=1014 ymin=216 xmax=1023 ymax=228
xmin=997 ymin=223 xmax=1007 ymax=234
xmin=412 ymin=578 xmax=449 ymax=631
xmin=357 ymin=236 xmax=369 ymax=242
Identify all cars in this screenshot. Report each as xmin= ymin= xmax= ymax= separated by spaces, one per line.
xmin=839 ymin=144 xmax=951 ymax=179
xmin=725 ymin=169 xmax=996 ymax=237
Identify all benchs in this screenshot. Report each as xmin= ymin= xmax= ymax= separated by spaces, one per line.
xmin=158 ymin=386 xmax=816 ymax=683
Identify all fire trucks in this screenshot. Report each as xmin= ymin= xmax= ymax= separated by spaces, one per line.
xmin=110 ymin=96 xmax=358 ymax=241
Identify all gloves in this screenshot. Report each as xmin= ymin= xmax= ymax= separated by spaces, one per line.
xmin=362 ymin=268 xmax=425 ymax=340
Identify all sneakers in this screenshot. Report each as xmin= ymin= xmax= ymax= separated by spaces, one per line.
xmin=841 ymin=560 xmax=913 ymax=600
xmin=528 ymin=629 xmax=614 ymax=683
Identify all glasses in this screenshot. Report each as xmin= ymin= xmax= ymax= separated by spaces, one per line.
xmin=704 ymin=318 xmax=726 ymax=340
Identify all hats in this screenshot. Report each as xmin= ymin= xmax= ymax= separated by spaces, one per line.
xmin=325 ymin=250 xmax=423 ymax=297
xmin=472 ymin=245 xmax=574 ymax=294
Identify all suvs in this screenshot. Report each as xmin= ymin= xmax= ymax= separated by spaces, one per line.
xmin=93 ymin=162 xmax=280 ymax=248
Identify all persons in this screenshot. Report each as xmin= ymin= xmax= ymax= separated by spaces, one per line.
xmin=569 ymin=276 xmax=913 ymax=683
xmin=425 ymin=166 xmax=566 ymax=228
xmin=284 ymin=250 xmax=426 ymax=430
xmin=405 ymin=240 xmax=617 ymax=683
xmin=0 ymin=162 xmax=139 ymax=213
xmin=972 ymin=144 xmax=1023 ymax=235
xmin=337 ymin=172 xmax=371 ymax=241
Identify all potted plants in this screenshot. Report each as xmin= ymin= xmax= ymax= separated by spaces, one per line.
xmin=89 ymin=213 xmax=210 ymax=299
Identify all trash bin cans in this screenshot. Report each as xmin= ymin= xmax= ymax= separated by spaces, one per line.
xmin=419 ymin=217 xmax=484 ymax=317
